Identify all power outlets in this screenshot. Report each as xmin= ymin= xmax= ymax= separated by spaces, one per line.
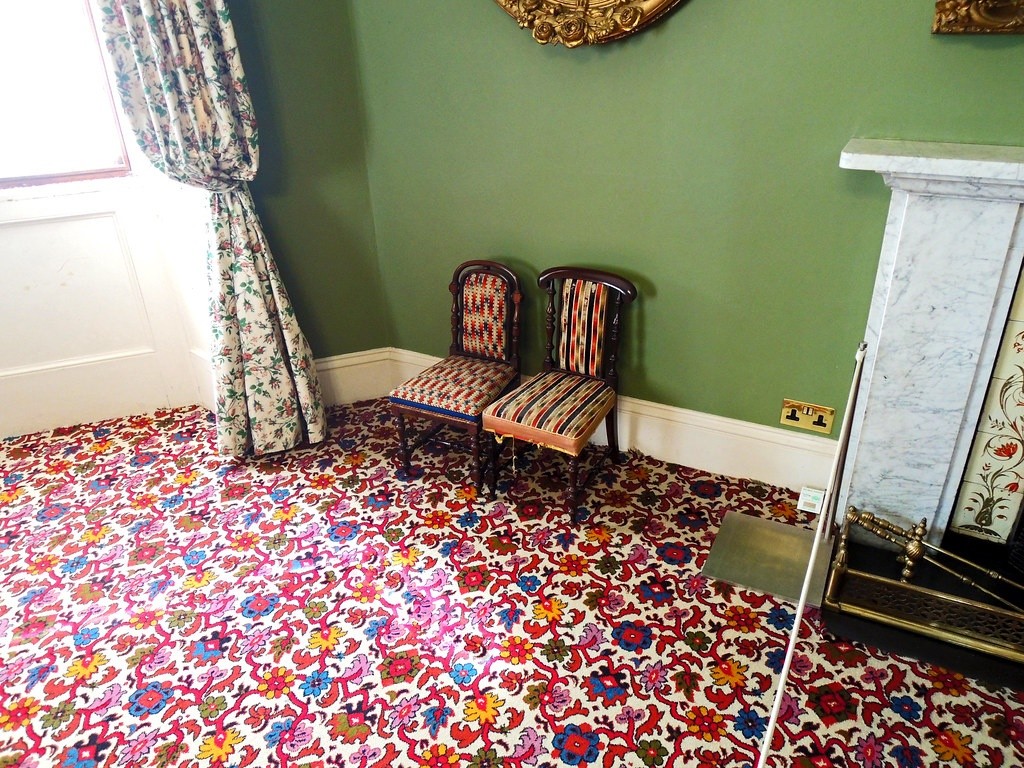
xmin=779 ymin=398 xmax=835 ymax=435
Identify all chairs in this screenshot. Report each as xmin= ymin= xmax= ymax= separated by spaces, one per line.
xmin=389 ymin=260 xmax=524 ymax=496
xmin=482 ymin=266 xmax=636 ymax=524
